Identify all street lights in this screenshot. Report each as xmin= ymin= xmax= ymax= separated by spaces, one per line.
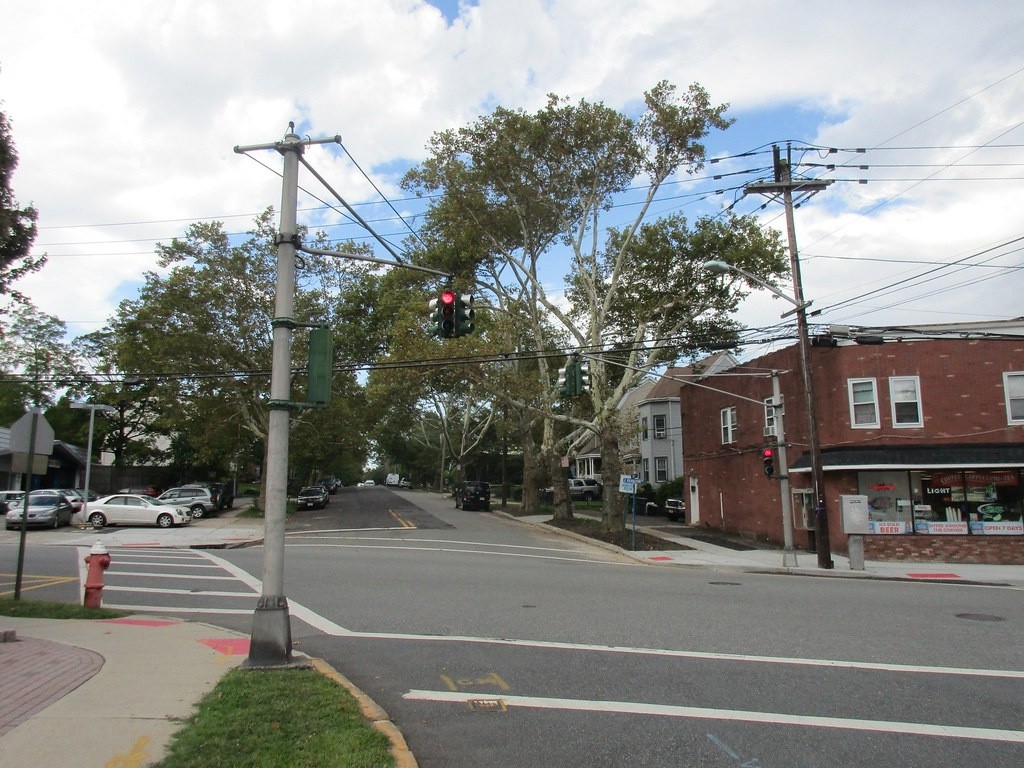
xmin=67 ymin=404 xmax=114 ymax=527
xmin=700 ymin=260 xmax=833 ymax=568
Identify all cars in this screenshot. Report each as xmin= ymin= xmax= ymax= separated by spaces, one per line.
xmin=356 ymin=479 xmax=375 ymax=488
xmin=212 ymin=482 xmax=234 ymax=510
xmin=303 ymin=476 xmax=343 ymax=504
xmin=664 ymin=496 xmax=686 ymax=521
xmin=399 ymin=478 xmax=414 ymax=489
xmin=81 ymin=493 xmax=193 ymax=529
xmin=0 ymin=485 xmax=163 ymax=514
xmin=6 ymin=494 xmax=74 ymax=530
xmin=296 ymin=488 xmax=326 ymax=510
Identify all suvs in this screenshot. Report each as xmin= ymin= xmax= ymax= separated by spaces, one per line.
xmin=538 ymin=478 xmax=603 ymax=502
xmin=142 ymin=482 xmax=223 ymax=520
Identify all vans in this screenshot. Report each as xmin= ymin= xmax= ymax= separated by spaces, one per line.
xmin=385 ymin=473 xmax=399 ymax=486
xmin=454 ymin=481 xmax=491 ymax=512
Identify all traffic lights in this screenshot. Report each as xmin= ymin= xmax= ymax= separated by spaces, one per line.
xmin=428 ymin=290 xmax=439 ymax=339
xmin=762 ymin=444 xmax=776 ymax=479
xmin=438 ymin=289 xmax=457 ymax=337
xmin=574 ymin=364 xmax=592 ymax=396
xmin=454 ymin=289 xmax=475 ymax=338
xmin=558 ymin=364 xmax=572 ymax=399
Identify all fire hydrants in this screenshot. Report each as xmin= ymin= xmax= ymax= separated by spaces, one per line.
xmin=82 ymin=539 xmax=112 ymax=609
